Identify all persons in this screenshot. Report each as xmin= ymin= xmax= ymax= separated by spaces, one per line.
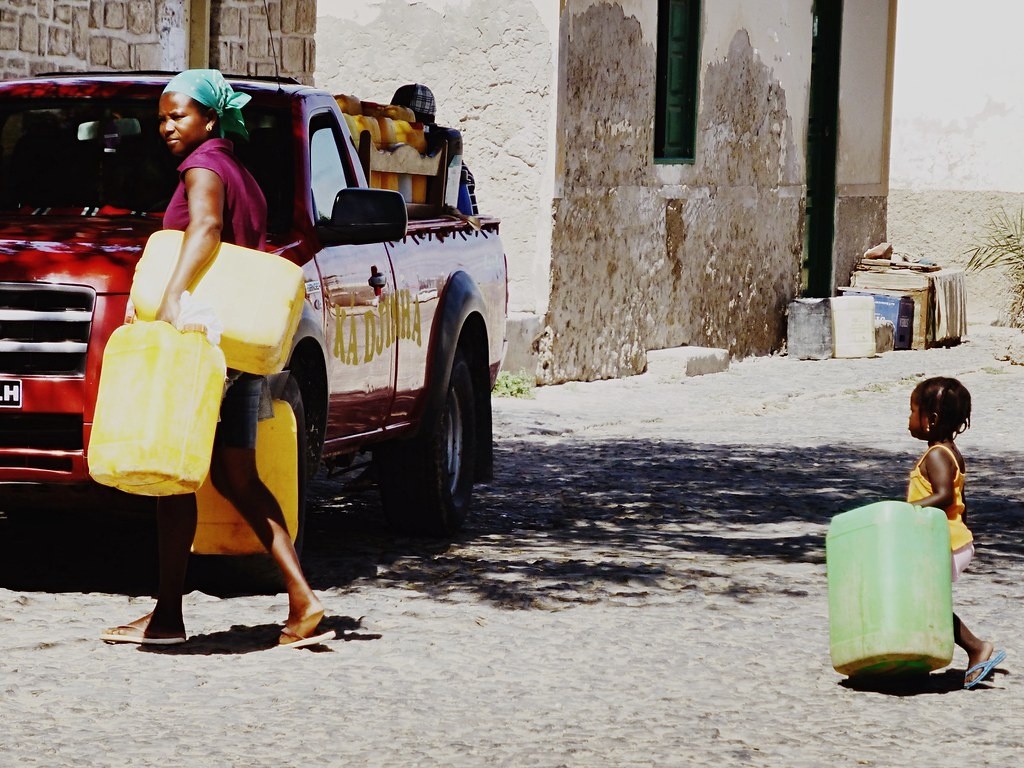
xmin=389 ymin=83 xmax=478 ymax=215
xmin=907 ymin=376 xmax=1006 ymax=689
xmin=102 ymin=68 xmax=337 ymax=648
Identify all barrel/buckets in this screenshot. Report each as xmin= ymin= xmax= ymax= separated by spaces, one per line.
xmin=87 ymin=316 xmax=228 ymax=497
xmin=830 ymin=296 xmax=877 ymax=358
xmin=191 ymin=400 xmax=300 ymax=556
xmin=827 ymin=501 xmax=956 ymax=678
xmin=788 ymin=299 xmax=833 ymax=359
xmin=124 ymin=230 xmax=307 ymax=376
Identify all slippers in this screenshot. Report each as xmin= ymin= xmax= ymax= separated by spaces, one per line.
xmin=259 ymin=626 xmax=336 ymax=649
xmin=963 ymin=652 xmax=1007 ymax=689
xmin=99 ymin=625 xmax=186 ymax=645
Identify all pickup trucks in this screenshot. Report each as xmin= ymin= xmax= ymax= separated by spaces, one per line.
xmin=0 ymin=73 xmax=509 ymax=596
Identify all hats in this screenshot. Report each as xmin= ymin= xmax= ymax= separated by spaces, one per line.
xmin=391 ymin=84 xmax=437 ymax=115
